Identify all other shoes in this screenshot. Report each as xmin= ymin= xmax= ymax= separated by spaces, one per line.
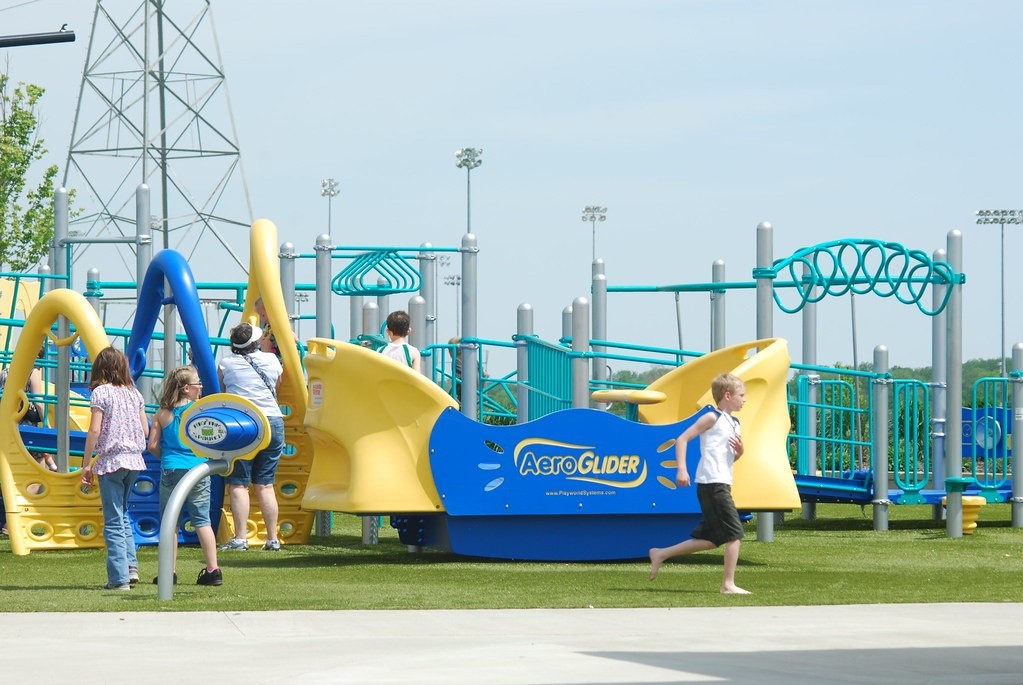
xmin=49 ymin=463 xmax=58 ymax=472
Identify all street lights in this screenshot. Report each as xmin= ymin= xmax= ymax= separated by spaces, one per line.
xmin=455 ymin=147 xmax=484 ymax=234
xmin=975 ymin=209 xmax=1023 ymax=376
xmin=582 ymin=205 xmax=608 ymax=261
xmin=321 ymin=178 xmax=341 ymax=234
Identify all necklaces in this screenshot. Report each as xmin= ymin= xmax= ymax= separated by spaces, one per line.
xmin=717 ymin=407 xmax=738 ymax=438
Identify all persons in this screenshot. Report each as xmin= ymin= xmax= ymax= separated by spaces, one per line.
xmin=217 ymin=322 xmax=284 ymax=551
xmin=254 ymin=296 xmax=283 ymax=368
xmin=448 ymin=337 xmax=490 ymax=412
xmin=649 ymin=371 xmax=752 ymax=594
xmin=376 ymin=311 xmax=421 ymax=373
xmin=0 ymin=346 xmax=58 ymax=536
xmin=148 ymin=366 xmax=223 ymax=585
xmin=82 ymin=345 xmax=150 ymax=590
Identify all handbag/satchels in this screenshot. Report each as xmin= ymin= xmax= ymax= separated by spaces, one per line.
xmin=20 ymin=397 xmax=41 ymax=423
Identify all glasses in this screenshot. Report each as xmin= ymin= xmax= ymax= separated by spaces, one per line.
xmin=187 ymin=381 xmax=202 ymax=388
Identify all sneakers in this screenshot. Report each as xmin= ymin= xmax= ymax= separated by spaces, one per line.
xmin=102 ymin=581 xmax=131 ymax=591
xmin=261 ymin=540 xmax=281 ymax=552
xmin=153 ymin=572 xmax=178 ymax=586
xmin=129 ymin=568 xmax=139 ymax=583
xmin=197 ymin=567 xmax=223 ymax=586
xmin=216 ymin=538 xmax=249 ymax=552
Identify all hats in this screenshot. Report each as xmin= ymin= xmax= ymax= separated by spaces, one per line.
xmin=231 ymin=322 xmax=263 ymax=349
